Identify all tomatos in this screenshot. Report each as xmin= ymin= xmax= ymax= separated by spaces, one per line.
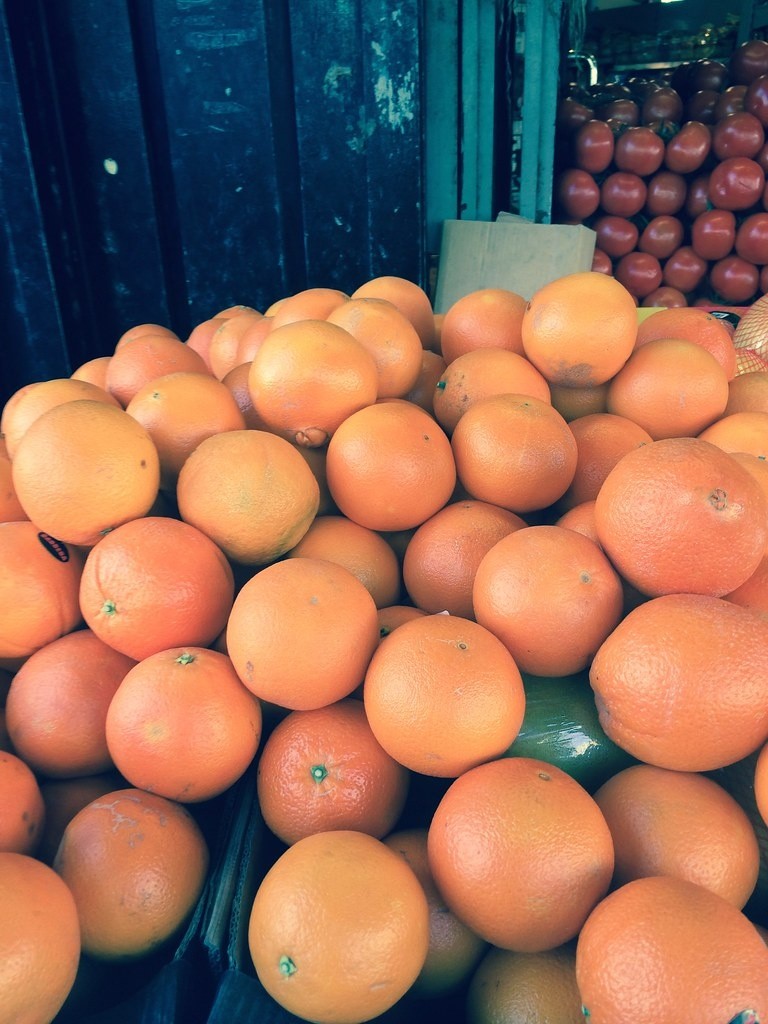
xmin=552 ymin=38 xmax=768 ymax=308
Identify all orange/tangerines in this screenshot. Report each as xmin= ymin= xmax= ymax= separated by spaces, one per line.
xmin=0 ymin=272 xmax=767 ymax=1024
